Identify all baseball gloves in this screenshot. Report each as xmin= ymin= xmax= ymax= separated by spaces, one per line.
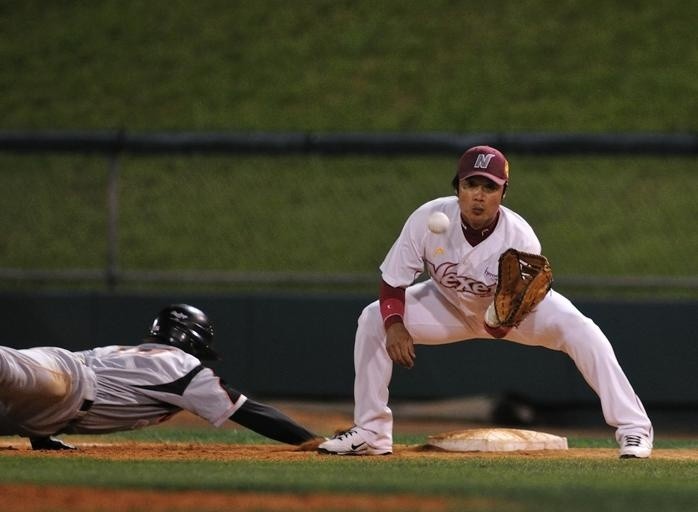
xmin=495 ymin=249 xmax=553 ymax=326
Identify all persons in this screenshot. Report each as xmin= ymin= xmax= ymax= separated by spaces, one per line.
xmin=317 ymin=146 xmax=655 ymax=459
xmin=1 ymin=304 xmax=352 ymax=452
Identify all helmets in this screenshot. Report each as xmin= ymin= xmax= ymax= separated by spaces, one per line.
xmin=149 ymin=302 xmax=213 ymax=359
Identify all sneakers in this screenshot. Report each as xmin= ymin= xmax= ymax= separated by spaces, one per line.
xmin=318 ymin=426 xmax=395 ymax=456
xmin=618 ymin=433 xmax=655 ymax=457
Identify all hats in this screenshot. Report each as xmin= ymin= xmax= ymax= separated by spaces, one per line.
xmin=457 ymin=145 xmax=510 ymax=186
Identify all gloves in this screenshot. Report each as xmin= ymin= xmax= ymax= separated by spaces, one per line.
xmin=30 ymin=436 xmax=79 ymax=451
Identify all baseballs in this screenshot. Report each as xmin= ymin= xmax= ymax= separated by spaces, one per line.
xmin=428 ymin=212 xmax=450 ymax=234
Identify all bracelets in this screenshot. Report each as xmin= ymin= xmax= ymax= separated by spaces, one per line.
xmin=380 ymin=298 xmax=403 ymax=321
xmin=484 ymin=301 xmax=503 ymax=329
